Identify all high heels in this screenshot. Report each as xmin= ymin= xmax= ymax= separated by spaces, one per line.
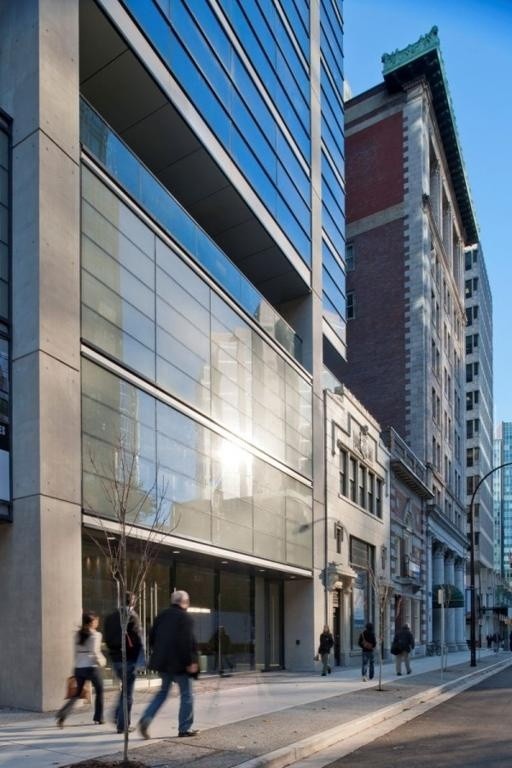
xmin=94 ymin=719 xmax=107 ymax=725
xmin=55 ymin=713 xmax=64 ymax=727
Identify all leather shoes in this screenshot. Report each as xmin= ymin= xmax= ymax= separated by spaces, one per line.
xmin=178 ymin=730 xmax=201 ymax=737
xmin=138 ymin=719 xmax=152 ymax=739
xmin=396 ymin=670 xmax=412 ymax=675
xmin=113 ymin=719 xmax=136 ymax=734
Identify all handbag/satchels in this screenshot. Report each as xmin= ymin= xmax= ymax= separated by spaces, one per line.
xmin=390 ymin=640 xmax=401 ymax=655
xmin=64 ymin=676 xmax=92 ymax=700
xmin=364 ymin=642 xmax=372 ymax=649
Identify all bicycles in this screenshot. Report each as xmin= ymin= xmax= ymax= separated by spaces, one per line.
xmin=427 ymin=638 xmax=441 ymax=656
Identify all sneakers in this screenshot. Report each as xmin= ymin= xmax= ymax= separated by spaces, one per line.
xmin=321 ymin=668 xmax=332 ymax=676
xmin=362 ymin=677 xmax=368 ymax=682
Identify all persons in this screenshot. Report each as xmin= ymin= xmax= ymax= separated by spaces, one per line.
xmin=392 ymin=623 xmax=415 ymax=676
xmin=134 ymin=590 xmax=202 ymax=738
xmin=211 ymin=626 xmax=237 ymax=672
xmin=485 ymin=631 xmax=493 ymax=651
xmin=317 ymin=624 xmax=335 ymax=676
xmin=103 ymin=590 xmax=145 ymax=734
xmin=54 ymin=611 xmax=109 ymax=730
xmin=493 ymin=630 xmax=502 ymax=654
xmin=357 ymin=623 xmax=377 ymax=682
